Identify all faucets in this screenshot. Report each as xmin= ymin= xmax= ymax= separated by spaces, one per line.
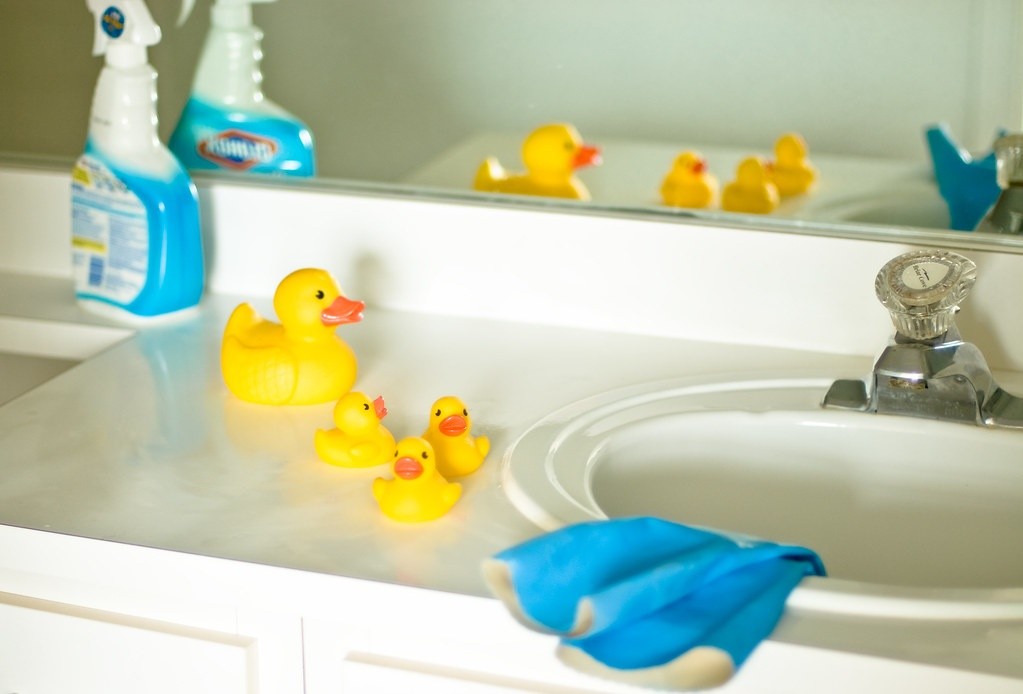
xmin=819 ymin=248 xmax=1023 ymax=431
xmin=987 ymin=133 xmax=1023 ymax=237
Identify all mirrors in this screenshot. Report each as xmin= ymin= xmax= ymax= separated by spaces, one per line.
xmin=0 ymin=0 xmax=1023 ymax=254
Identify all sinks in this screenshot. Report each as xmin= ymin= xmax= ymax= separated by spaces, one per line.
xmin=808 ymin=188 xmax=986 ymax=231
xmin=505 ymin=378 xmax=1023 ymax=624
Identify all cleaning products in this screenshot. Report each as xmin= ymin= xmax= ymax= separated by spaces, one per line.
xmin=167 ymin=0 xmax=317 ymax=179
xmin=69 ymin=0 xmax=205 ymax=318
